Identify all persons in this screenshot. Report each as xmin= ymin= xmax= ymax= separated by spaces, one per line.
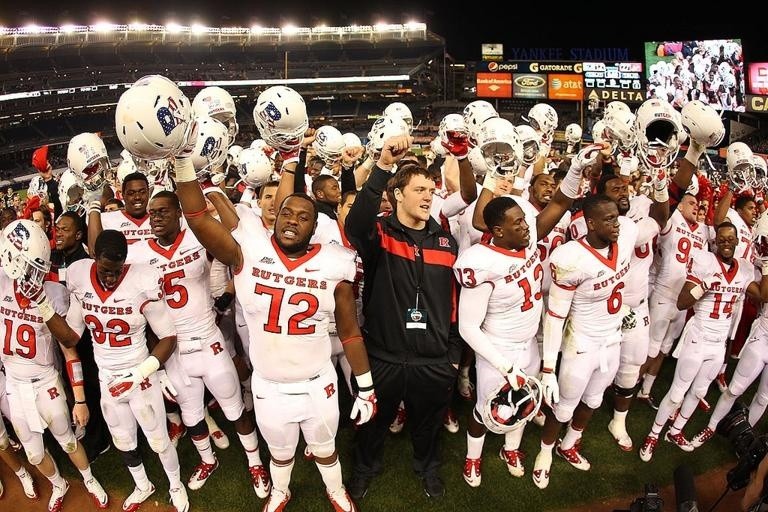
xmin=166 ymin=121 xmax=378 ymax=510
xmin=699 ymin=199 xmax=710 ymax=216
xmin=23 ymin=230 xmax=190 ymax=512
xmin=0 ymin=260 xmax=109 ymax=512
xmin=80 ymin=176 xmax=273 ymax=499
xmin=377 ymin=186 xmax=396 ymax=219
xmin=0 ymin=162 xmax=63 ymax=250
xmin=532 ymin=152 xmax=671 ymax=489
xmin=639 ymin=220 xmax=768 ymax=463
xmin=197 ymin=174 xmax=281 ymax=363
xmin=84 ymin=172 xmax=240 ymax=452
xmin=224 ymin=174 xmax=244 ymax=204
xmin=362 ymin=129 xmax=477 ymax=430
xmin=344 ymin=135 xmax=459 ymax=502
xmin=696 ymin=205 xmax=706 ymax=224
xmin=713 ymin=174 xmax=759 ymax=396
xmin=740 ymin=452 xmax=768 ymax=512
xmin=647 ymin=39 xmax=745 ymax=113
xmin=264 ymin=146 xmax=286 ymax=187
xmin=631 ymin=194 xmax=732 ymax=410
xmin=182 ymin=169 xmax=260 ymax=418
xmin=295 ymin=127 xmax=361 ymax=222
xmin=0 ymin=370 xmax=40 ymax=501
xmin=690 ymin=302 xmax=768 ymax=450
xmin=523 ymin=172 xmax=573 ymax=428
xmin=274 ymin=134 xmax=366 ymax=460
xmin=454 ymin=153 xmax=515 ymax=400
xmin=43 ymin=210 xmax=112 ymax=472
xmin=569 ymin=133 xmax=716 ymax=452
xmin=452 ymin=144 xmax=608 ymax=488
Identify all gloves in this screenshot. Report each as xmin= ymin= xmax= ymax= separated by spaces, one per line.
xmin=349 ymin=393 xmax=377 ymax=425
xmin=157 ymin=369 xmax=178 ymax=402
xmin=650 ymin=167 xmax=667 ymax=191
xmin=107 ymin=368 xmax=144 ymax=400
xmin=441 ymin=129 xmax=468 ymax=160
xmin=85 ymin=179 xmax=103 ymax=206
xmin=504 ymin=364 xmax=529 ymax=392
xmin=542 ymin=368 xmax=560 ymax=404
xmin=754 ymin=235 xmax=768 ymax=261
xmin=23 ymin=274 xmax=46 ymax=304
xmin=700 ymin=272 xmax=723 ymax=293
xmin=570 ymin=143 xmax=604 ymax=175
xmin=279 ymin=137 xmax=299 ymax=163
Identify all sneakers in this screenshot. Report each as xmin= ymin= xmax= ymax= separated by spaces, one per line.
xmin=209 ymin=427 xmax=230 ymax=449
xmin=462 ymin=455 xmax=482 ymax=487
xmin=262 ymin=489 xmax=292 ymax=512
xmin=457 ymin=374 xmax=473 ymax=401
xmin=348 ymin=474 xmax=370 ymax=500
xmin=122 ymin=481 xmax=156 ymax=512
xmin=388 ymin=407 xmax=408 ymax=433
xmin=188 ymin=451 xmax=220 ymax=491
xmin=18 ymin=472 xmax=40 ymax=499
xmin=326 ymin=483 xmax=360 ymax=512
xmin=83 ymin=475 xmax=110 ymax=509
xmin=498 ymin=443 xmax=526 ymax=478
xmin=566 ymin=420 xmax=583 ymax=453
xmin=169 ymin=481 xmax=190 ymax=512
xmin=697 ymin=398 xmax=711 ymax=413
xmin=607 ymin=418 xmax=634 ymax=452
xmin=244 ymin=393 xmax=253 ymax=412
xmin=555 ymin=443 xmax=591 ymax=471
xmin=168 ymin=421 xmax=188 ymax=449
xmin=442 ymin=407 xmax=460 ymax=434
xmin=638 ymin=435 xmax=659 ymax=462
xmin=420 ymin=476 xmax=446 ymax=497
xmin=635 ymin=387 xmax=659 ymax=410
xmin=71 ymin=437 xmax=110 ymax=471
xmin=663 ymin=429 xmax=695 ymax=452
xmin=47 ymin=477 xmax=71 ymax=512
xmin=532 ymin=453 xmax=553 ymax=490
xmin=715 ymin=373 xmax=728 ymax=393
xmin=689 ymin=426 xmax=715 ymax=448
xmin=208 ymin=397 xmax=220 ymax=409
xmin=247 ymin=463 xmax=271 ymax=499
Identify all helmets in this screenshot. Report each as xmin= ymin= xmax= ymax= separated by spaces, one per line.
xmin=27 ymin=176 xmax=48 ymax=201
xmin=482 ymin=375 xmax=543 ymax=434
xmin=364 ymin=115 xmax=410 ymax=162
xmin=312 ymin=125 xmax=346 ymax=165
xmin=528 ymin=102 xmax=558 ymax=140
xmin=117 ymin=160 xmax=160 ymax=189
xmin=467 ymin=146 xmax=487 ymax=175
xmin=744 ymin=155 xmax=767 ymax=193
xmin=726 ymin=141 xmax=757 ymax=191
xmin=193 ymin=86 xmax=239 ymax=148
xmin=477 ymin=117 xmax=523 ymax=178
xmin=236 ymin=148 xmax=271 ymax=188
xmin=634 ymin=100 xmax=680 ymax=170
xmin=516 ymin=124 xmax=542 ymax=165
xmin=618 ymin=156 xmax=639 ymax=174
xmin=438 ymin=113 xmax=465 ymax=143
xmin=681 ymin=100 xmax=726 ymax=147
xmin=565 ymin=122 xmax=582 ymax=144
xmin=673 ymin=110 xmax=687 ymax=145
xmin=67 ymin=132 xmax=111 ymax=192
xmin=190 ymin=115 xmax=228 ymax=180
xmin=463 ymin=100 xmax=499 ymax=147
xmin=227 ymin=145 xmax=243 ymax=167
xmin=115 ymin=75 xmax=194 ymax=161
xmin=253 ymin=85 xmax=309 ymax=153
xmin=382 ymin=101 xmax=413 ymax=135
xmin=591 ymin=120 xmax=619 ymax=156
xmin=603 ymin=100 xmax=631 ymax=117
xmin=429 ymin=136 xmax=447 ymax=156
xmin=0 ymin=219 xmax=52 ymax=299
xmin=57 ymin=169 xmax=91 ymax=217
xmin=604 ymin=110 xmax=638 ymax=152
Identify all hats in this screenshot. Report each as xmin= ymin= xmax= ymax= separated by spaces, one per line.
xmin=32 ymin=146 xmax=48 ymax=173
